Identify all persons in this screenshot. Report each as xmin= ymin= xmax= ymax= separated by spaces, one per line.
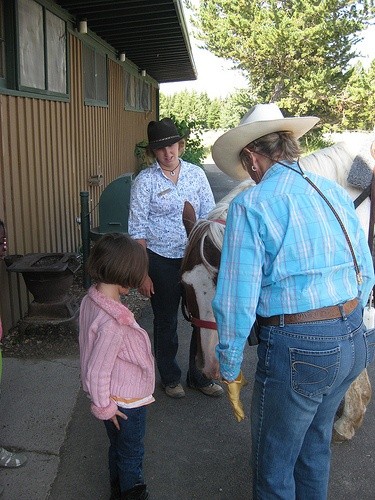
xmin=0 ymin=447 xmax=27 ymax=467
xmin=212 ymin=103 xmax=375 ymax=500
xmin=77 ymin=232 xmax=155 ymax=500
xmin=127 ymin=117 xmax=225 ymax=399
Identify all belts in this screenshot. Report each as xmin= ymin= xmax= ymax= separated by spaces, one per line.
xmin=258 ymin=297 xmax=359 ymax=326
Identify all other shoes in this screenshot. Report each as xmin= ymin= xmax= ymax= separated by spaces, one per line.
xmin=1 ymin=449 xmax=28 ymax=468
xmin=161 ymin=380 xmax=185 ymax=398
xmin=188 ymin=377 xmax=225 ymax=397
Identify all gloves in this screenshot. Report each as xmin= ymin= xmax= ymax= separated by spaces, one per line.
xmin=221 ymin=373 xmax=249 ymax=423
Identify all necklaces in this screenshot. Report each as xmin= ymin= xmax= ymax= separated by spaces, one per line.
xmin=161 ymin=164 xmax=180 ymax=176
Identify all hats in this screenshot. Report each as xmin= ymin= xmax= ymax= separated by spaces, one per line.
xmin=211 ymin=103 xmax=320 ymax=179
xmin=137 ymin=118 xmax=187 ymax=150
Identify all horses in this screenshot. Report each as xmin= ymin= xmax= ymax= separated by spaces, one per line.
xmin=181 ymin=128 xmax=375 ymax=449
